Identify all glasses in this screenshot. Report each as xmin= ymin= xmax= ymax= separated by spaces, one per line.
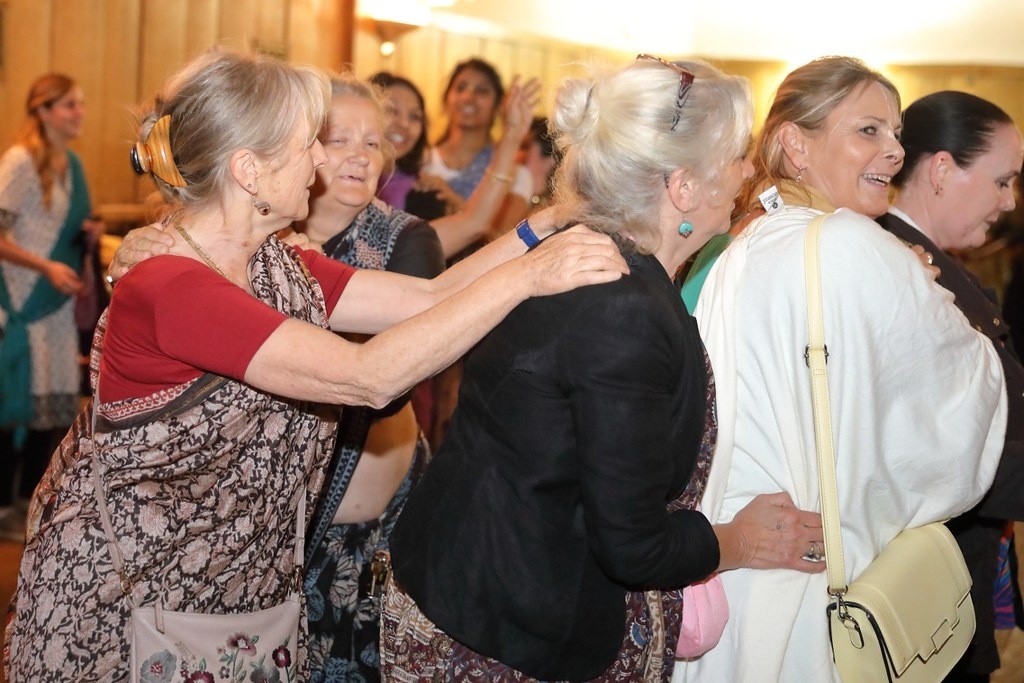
xmin=635 ymin=53 xmax=696 ymax=133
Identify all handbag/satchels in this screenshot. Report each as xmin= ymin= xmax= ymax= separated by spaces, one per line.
xmin=826 ymin=522 xmax=977 ymax=683
xmin=118 ymin=567 xmax=301 ymax=683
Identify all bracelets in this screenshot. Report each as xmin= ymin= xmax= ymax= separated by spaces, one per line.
xmin=515 ymin=218 xmax=542 ymax=249
xmin=484 ymin=167 xmax=517 ymax=184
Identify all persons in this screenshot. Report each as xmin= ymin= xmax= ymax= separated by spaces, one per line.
xmin=0 ymin=51 xmax=1024 ymax=682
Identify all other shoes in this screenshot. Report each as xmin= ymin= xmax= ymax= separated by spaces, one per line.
xmin=0 ymin=498 xmax=31 ymax=544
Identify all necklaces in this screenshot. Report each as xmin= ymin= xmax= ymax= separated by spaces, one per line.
xmin=173 ymin=221 xmax=231 ymax=280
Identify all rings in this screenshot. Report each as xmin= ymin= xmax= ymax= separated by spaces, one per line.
xmin=808 ymin=540 xmax=822 ymax=560
xmin=106 ymin=271 xmax=117 ymax=285
xmin=926 ymin=254 xmax=933 ymax=265
